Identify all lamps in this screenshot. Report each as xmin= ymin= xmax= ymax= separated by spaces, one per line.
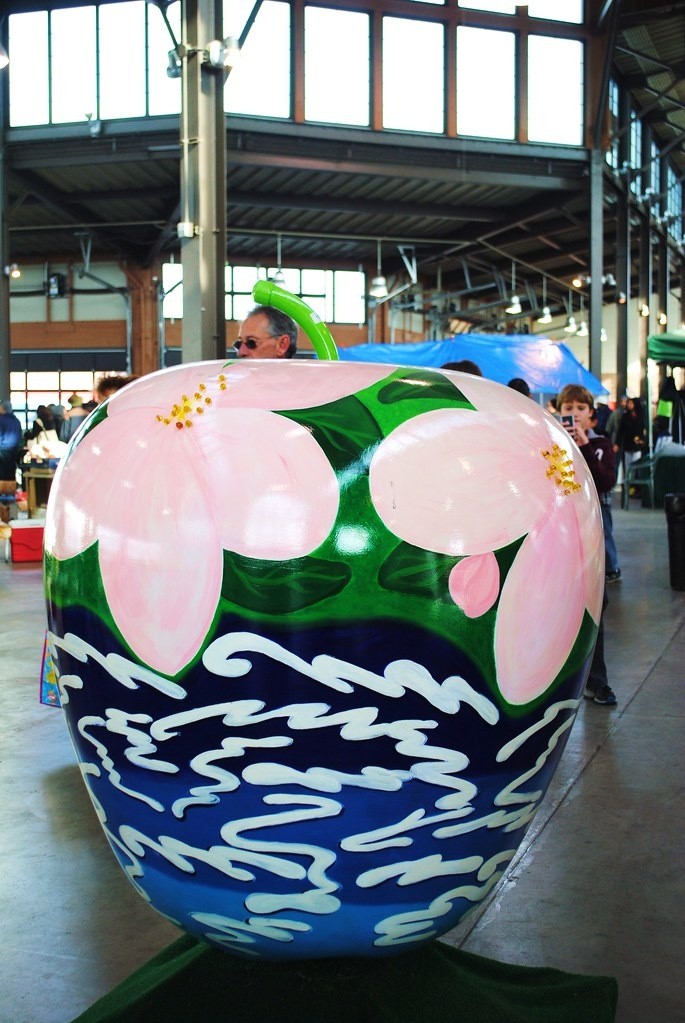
xmin=506 ymin=262 xmax=607 ymax=342
xmin=370 ymin=241 xmax=387 ymax=297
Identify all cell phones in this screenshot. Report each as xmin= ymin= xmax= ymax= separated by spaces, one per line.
xmin=562 ymin=415 xmax=576 ymax=439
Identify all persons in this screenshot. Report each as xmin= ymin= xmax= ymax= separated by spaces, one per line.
xmin=236 ymin=305 xmax=297 ymax=360
xmin=444 ymin=361 xmax=645 ymax=704
xmin=0 ymin=374 xmax=142 ymax=508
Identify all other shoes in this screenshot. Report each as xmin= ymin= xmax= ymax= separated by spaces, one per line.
xmin=605 ymin=568 xmax=621 ymax=583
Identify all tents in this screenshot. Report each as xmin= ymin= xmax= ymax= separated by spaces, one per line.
xmin=646 ymin=327 xmax=685 ymax=508
xmin=318 ymin=334 xmax=606 ymax=408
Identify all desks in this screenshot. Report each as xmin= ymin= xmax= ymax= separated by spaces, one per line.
xmin=22 ymin=471 xmax=54 ymax=518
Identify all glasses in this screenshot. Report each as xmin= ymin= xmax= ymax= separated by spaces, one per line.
xmin=233 ymin=332 xmax=284 ymax=350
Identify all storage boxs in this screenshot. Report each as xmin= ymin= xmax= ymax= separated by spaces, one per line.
xmin=9 ymin=519 xmax=44 ymax=562
xmin=31 ymin=458 xmax=61 ymax=470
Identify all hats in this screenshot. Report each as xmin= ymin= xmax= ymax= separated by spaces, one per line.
xmin=2 ymin=401 xmax=12 ymax=414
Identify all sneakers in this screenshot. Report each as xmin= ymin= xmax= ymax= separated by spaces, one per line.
xmin=583 ymin=684 xmax=617 ymax=706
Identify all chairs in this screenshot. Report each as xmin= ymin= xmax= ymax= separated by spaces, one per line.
xmin=621 ymin=453 xmax=656 ymax=510
